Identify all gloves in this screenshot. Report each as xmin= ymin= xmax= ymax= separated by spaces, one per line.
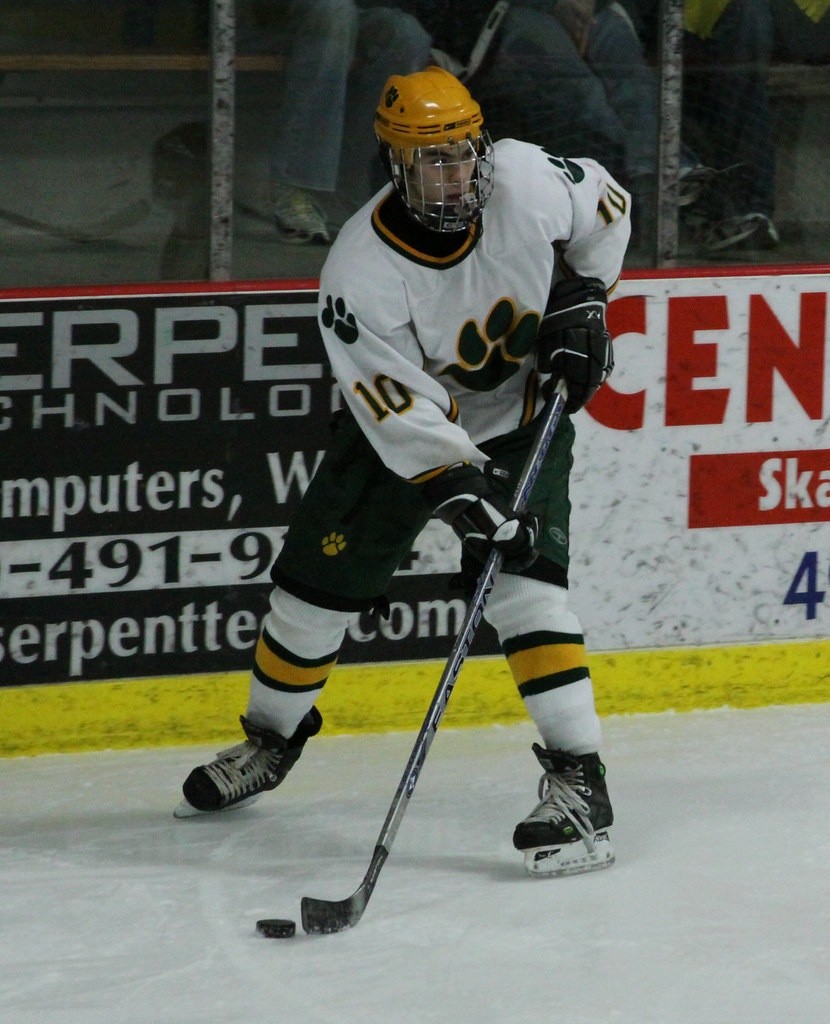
xmin=536 ymin=276 xmax=613 ymax=416
xmin=416 ymin=464 xmax=539 ymax=574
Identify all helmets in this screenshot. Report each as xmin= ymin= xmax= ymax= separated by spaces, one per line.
xmin=375 ymin=66 xmax=484 ymax=170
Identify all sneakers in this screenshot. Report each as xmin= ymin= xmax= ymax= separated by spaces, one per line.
xmin=641 ymin=212 xmax=778 ymax=260
xmin=635 ymin=162 xmax=747 ymax=212
xmin=173 ymin=705 xmax=323 ymax=819
xmin=254 ymin=179 xmax=332 ymax=246
xmin=512 ymin=742 xmax=616 ymax=878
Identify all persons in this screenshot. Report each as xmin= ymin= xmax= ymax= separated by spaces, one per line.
xmin=173 ymin=66 xmax=633 ymax=874
xmin=244 ymin=0 xmax=830 ymax=255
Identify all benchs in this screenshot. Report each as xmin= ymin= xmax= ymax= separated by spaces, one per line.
xmin=0 ymin=52 xmax=830 ymax=99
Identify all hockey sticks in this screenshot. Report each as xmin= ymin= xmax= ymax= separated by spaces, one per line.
xmin=300 ymin=376 xmax=572 ymax=937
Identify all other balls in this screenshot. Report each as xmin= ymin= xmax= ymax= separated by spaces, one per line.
xmin=255 ymin=918 xmax=296 ymax=938
